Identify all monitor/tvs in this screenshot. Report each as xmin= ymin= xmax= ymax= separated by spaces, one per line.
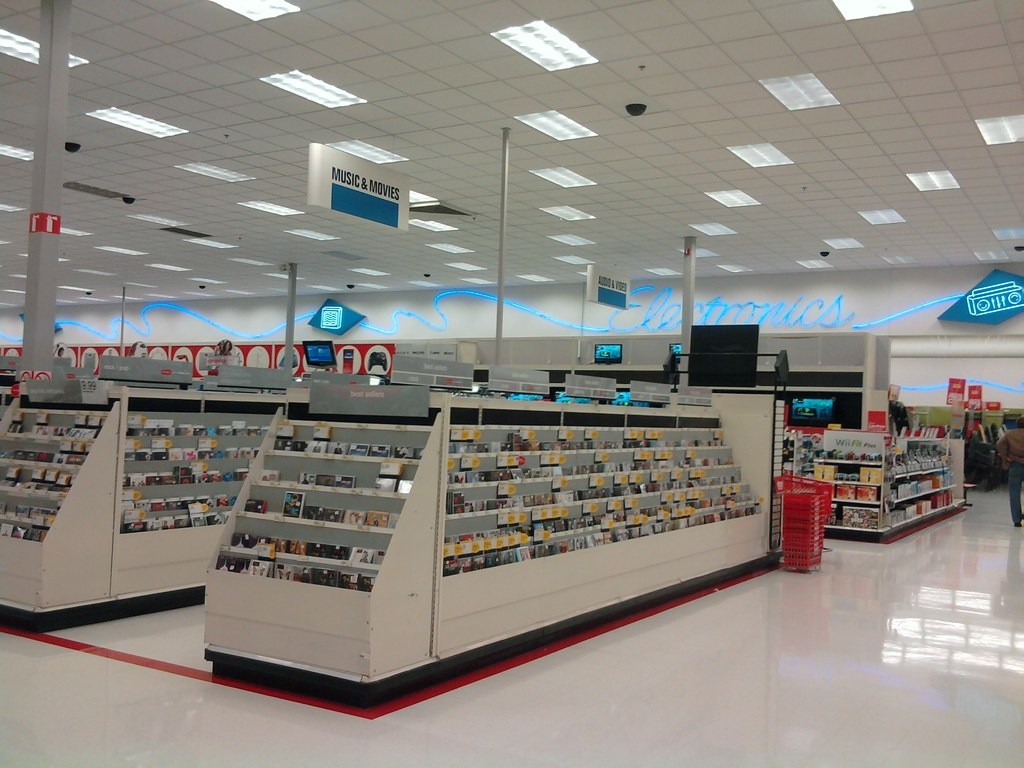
xmin=669 ymin=343 xmax=681 ymax=363
xmin=555 ymin=390 xmax=591 ymax=404
xmin=594 ymin=343 xmax=622 ymax=364
xmin=507 ymin=393 xmax=543 ymax=401
xmin=793 ymin=398 xmax=834 ymax=420
xmin=302 ymin=340 xmax=337 ymax=366
xmin=612 ymin=391 xmax=649 ymax=408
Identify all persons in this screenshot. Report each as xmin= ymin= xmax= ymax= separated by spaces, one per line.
xmin=996 ymin=417 xmax=1024 ymax=527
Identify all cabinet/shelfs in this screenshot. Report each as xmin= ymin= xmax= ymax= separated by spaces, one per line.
xmin=204 ymin=407 xmax=443 ymax=708
xmin=429 ymin=394 xmax=767 ymax=673
xmin=109 ymin=387 xmax=287 ymax=622
xmin=0 ymin=397 xmax=121 ymax=632
xmin=880 ymin=467 xmax=966 ymax=532
xmin=813 ymin=458 xmax=883 ymax=533
xmin=783 ymin=432 xmax=825 ymax=478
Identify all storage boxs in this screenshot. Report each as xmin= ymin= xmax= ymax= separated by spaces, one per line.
xmin=781 ymin=456 xmax=954 ymax=568
xmin=0 ymin=423 xmax=763 ymax=595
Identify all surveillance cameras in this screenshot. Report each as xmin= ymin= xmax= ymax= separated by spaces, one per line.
xmin=820 ymin=252 xmax=830 ymax=256
xmin=199 ymin=285 xmax=206 ymax=289
xmin=122 ymin=197 xmax=135 ymax=204
xmin=424 ymin=274 xmax=431 ymax=277
xmin=625 ymin=104 xmax=647 ymax=116
xmin=1014 ymin=246 xmax=1024 ymax=252
xmin=65 ymin=142 xmax=81 ymax=153
xmin=347 ymin=284 xmax=355 ymax=289
xmin=86 ymin=292 xmax=92 ymax=295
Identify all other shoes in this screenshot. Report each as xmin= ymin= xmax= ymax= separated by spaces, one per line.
xmin=1015 ymin=522 xmax=1022 ymax=527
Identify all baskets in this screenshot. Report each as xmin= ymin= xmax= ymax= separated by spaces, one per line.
xmin=783 ymin=493 xmax=825 ymax=571
